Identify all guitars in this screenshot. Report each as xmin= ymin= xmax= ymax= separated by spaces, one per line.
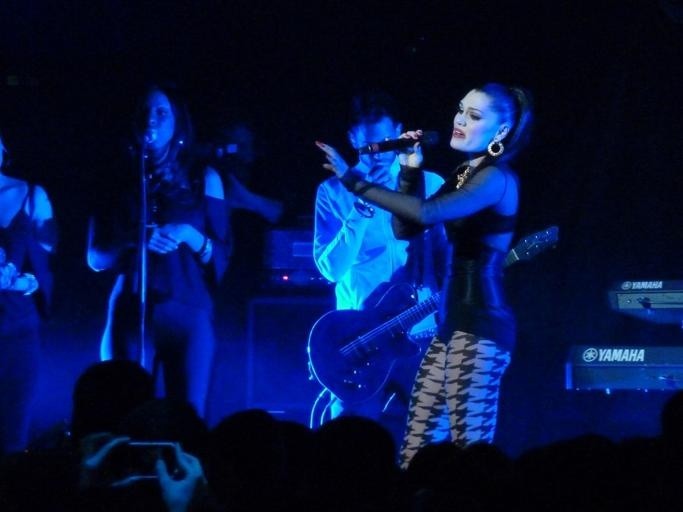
xmin=308 ymin=225 xmax=561 ymax=404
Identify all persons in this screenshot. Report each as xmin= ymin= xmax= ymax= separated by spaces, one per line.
xmin=317 ymin=81 xmax=535 ymax=468
xmin=0 ymin=134 xmax=62 ymax=454
xmin=2 ymin=361 xmax=682 ymax=510
xmin=87 ymin=76 xmax=236 ymax=419
xmin=314 ymin=94 xmax=448 ymax=309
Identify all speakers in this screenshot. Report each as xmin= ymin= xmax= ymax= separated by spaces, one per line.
xmin=242 ymin=289 xmax=336 ymax=425
xmin=262 ymin=213 xmax=335 ymax=289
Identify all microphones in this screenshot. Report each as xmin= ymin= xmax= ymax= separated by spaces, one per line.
xmin=143 ymin=131 xmax=156 ymax=143
xmin=358 ymin=130 xmax=439 ymax=155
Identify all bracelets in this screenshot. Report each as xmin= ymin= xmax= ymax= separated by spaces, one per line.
xmin=198 ymin=235 xmax=210 ymax=259
xmin=24 ymin=271 xmax=36 ymax=298
xmin=354 ymin=201 xmax=374 ymax=218
xmin=353 ymin=182 xmax=374 ymax=196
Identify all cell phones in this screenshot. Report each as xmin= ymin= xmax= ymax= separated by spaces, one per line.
xmin=105 ymin=443 xmax=184 ymax=480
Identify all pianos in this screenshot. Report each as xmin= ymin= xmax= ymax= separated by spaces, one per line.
xmin=565 ymin=345 xmax=682 ymax=391
xmin=608 ymin=279 xmax=682 ymax=325
